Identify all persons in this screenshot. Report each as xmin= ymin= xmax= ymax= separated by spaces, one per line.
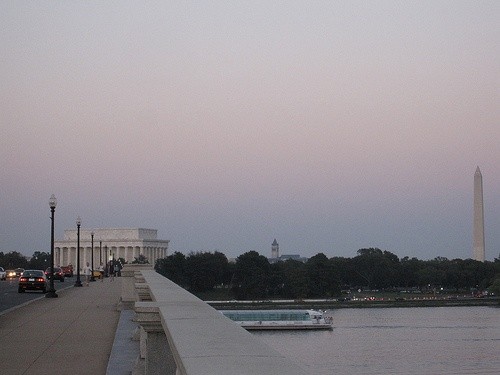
xmin=108 ymin=261 xmax=119 ymax=280
xmin=83 ymin=262 xmax=93 ymax=285
xmin=99 ymin=263 xmax=105 ymax=277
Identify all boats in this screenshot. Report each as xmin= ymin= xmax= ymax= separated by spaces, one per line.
xmin=216 ymin=308 xmax=333 ymax=331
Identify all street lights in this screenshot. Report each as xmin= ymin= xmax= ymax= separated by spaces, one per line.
xmin=74 ymin=215 xmax=82 ymax=287
xmin=89 ymin=231 xmax=96 ymax=282
xmin=99 ymin=238 xmax=114 ymax=278
xmin=45 ymin=193 xmax=58 ymax=298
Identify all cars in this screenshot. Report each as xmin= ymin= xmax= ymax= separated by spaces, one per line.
xmin=0 ymin=267 xmax=7 ymax=280
xmin=18 ymin=269 xmax=50 ymax=294
xmin=60 ymin=266 xmax=73 ymax=278
xmin=44 ymin=267 xmax=64 ymax=282
xmin=4 ymin=268 xmax=24 ymax=278
